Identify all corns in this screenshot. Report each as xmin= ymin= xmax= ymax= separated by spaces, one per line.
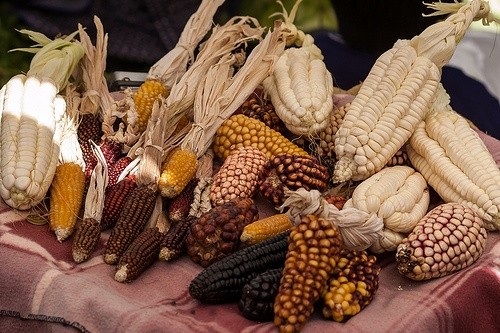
xmin=0 ymin=0 xmax=500 ymax=333
